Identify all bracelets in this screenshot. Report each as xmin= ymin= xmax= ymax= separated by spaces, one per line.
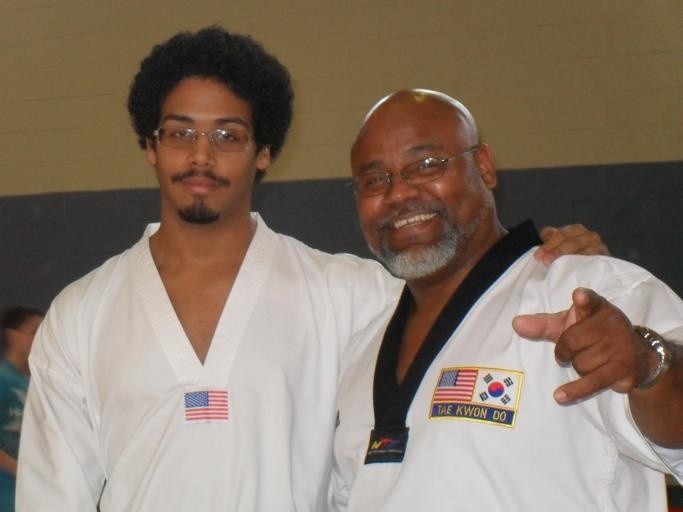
xmin=634 ymin=326 xmax=673 ymax=387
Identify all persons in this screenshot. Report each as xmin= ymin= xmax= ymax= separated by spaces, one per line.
xmin=326 ymin=88 xmax=682 ymax=512
xmin=0 ymin=306 xmax=45 ymax=512
xmin=15 ymin=26 xmax=610 ymax=512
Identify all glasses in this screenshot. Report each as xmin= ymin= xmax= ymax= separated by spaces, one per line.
xmin=346 ymin=147 xmax=477 ymax=197
xmin=146 ymin=126 xmax=265 ymax=152
